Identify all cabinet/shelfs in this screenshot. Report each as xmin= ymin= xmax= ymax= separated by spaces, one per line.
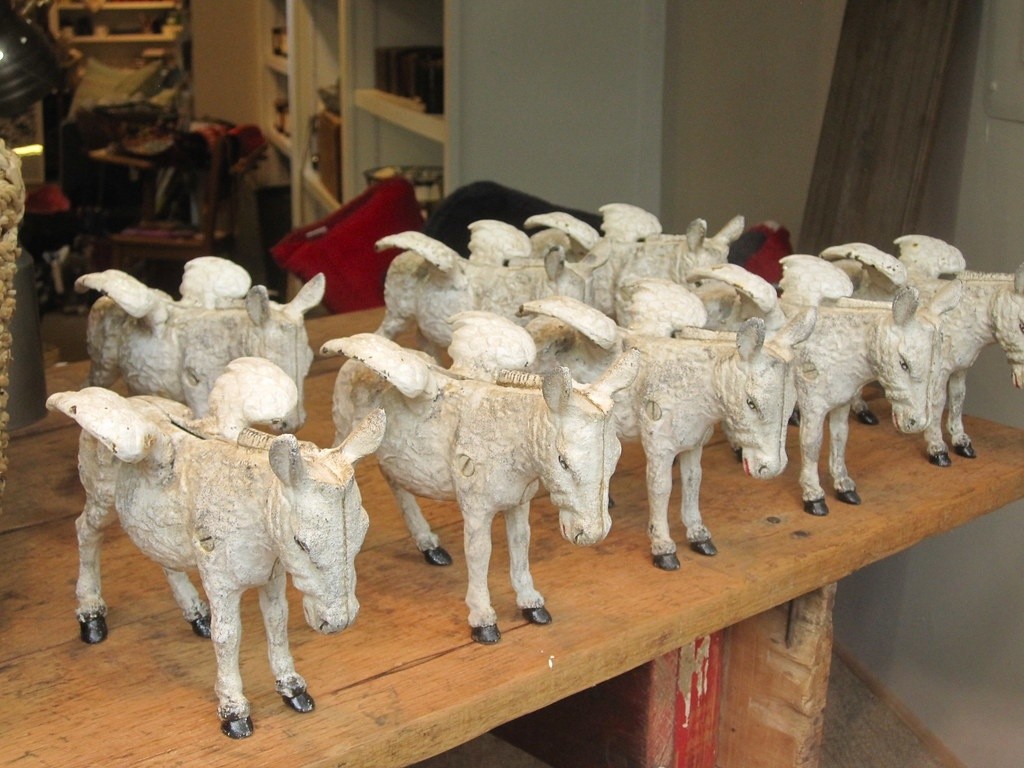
xmin=261 ymin=2 xmax=664 ymax=315
xmin=49 ymin=3 xmax=184 ymax=44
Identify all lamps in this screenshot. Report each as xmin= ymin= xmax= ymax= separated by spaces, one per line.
xmin=0 ymin=0 xmax=62 ymax=120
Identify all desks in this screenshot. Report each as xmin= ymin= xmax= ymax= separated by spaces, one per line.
xmin=1 ymin=304 xmax=1023 ymax=768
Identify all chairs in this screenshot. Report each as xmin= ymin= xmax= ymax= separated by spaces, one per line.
xmin=108 ymin=133 xmax=241 ymax=285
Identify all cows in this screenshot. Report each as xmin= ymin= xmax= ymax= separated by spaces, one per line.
xmin=45 ymin=199 xmax=1024 ymax=742
